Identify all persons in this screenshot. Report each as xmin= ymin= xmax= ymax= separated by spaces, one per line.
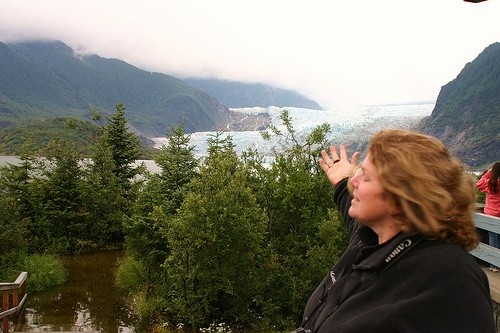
xmin=300 ymin=125 xmax=495 ymax=333
xmin=475 ymin=160 xmax=500 ymax=271
